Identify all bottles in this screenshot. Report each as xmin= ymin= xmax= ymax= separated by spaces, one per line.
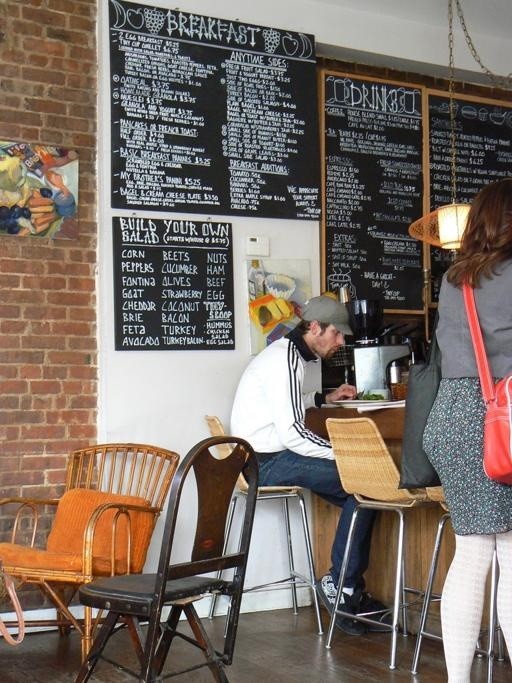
xmin=247 ymin=259 xmax=264 ymax=304
xmin=332 ymin=287 xmax=356 ymax=386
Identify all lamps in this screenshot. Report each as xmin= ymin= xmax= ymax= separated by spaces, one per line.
xmin=436 ymin=1 xmax=473 ymax=249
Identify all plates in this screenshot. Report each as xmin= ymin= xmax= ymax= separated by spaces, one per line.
xmin=333 ymin=399 xmax=389 ymax=409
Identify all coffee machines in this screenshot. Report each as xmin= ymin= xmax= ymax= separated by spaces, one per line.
xmin=346 ymin=299 xmax=413 ymax=403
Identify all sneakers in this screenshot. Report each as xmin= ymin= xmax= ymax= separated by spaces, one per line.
xmin=316 ymin=574 xmax=367 ymax=637
xmin=357 ymin=592 xmax=394 ymax=633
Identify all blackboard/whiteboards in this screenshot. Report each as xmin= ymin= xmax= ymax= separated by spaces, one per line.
xmin=320 ymin=71 xmax=426 ymax=314
xmin=428 ymin=90 xmax=512 ymax=308
xmin=112 ymin=216 xmax=236 ymax=352
xmin=107 ymin=0 xmax=322 ymax=222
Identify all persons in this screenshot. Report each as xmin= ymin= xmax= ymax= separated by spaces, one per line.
xmin=231 ymin=296 xmax=394 ymax=638
xmin=422 ymin=178 xmax=511 ymax=682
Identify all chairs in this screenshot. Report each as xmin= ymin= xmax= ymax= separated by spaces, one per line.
xmin=326 ymin=417 xmax=442 ymax=670
xmin=0 ymin=443 xmax=180 ymax=666
xmin=203 ymin=416 xmax=323 ymax=635
xmin=410 ymin=485 xmax=504 ymax=683
xmin=73 ymin=437 xmax=259 ymax=683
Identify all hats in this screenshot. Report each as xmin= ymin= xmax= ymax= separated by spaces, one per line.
xmin=301 ymin=296 xmax=355 ymax=337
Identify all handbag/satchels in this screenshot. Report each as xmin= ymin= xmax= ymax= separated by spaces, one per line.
xmin=481 ymin=374 xmax=512 ymax=486
xmin=395 ymin=362 xmax=443 ymax=490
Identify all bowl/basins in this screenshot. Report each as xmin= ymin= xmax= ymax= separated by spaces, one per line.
xmin=265 ymin=274 xmax=296 ymax=300
